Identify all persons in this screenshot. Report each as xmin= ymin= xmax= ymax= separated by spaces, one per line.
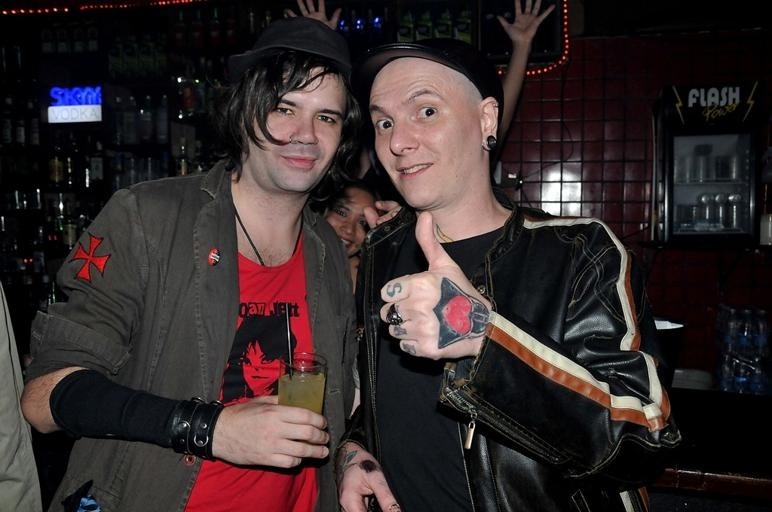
xmin=324 ymin=169 xmax=403 ymax=295
xmin=333 ymin=35 xmax=683 ymax=512
xmin=20 ymin=15 xmax=359 ymax=512
xmin=284 ymin=0 xmax=558 ymax=159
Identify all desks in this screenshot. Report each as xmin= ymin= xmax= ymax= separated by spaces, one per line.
xmin=629 ymin=386 xmax=767 ymax=512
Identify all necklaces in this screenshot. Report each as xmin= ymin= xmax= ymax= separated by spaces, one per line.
xmin=234 ymin=204 xmax=305 ymax=268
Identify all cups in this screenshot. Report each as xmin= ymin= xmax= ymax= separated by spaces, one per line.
xmin=278 ymin=352 xmax=327 ymax=414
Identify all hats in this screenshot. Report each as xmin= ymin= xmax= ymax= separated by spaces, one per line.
xmin=353 ymin=38 xmax=505 ymax=130
xmin=230 ymin=17 xmax=355 ymax=81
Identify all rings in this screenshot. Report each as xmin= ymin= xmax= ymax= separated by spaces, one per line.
xmin=387 ymin=301 xmax=402 ymax=325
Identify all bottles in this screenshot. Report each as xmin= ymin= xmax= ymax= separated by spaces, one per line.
xmin=674 ymin=132 xmax=749 ymax=233
xmin=714 ymin=304 xmax=767 ymax=364
xmin=719 ymin=356 xmax=767 ymax=396
xmin=0 ymin=81 xmax=208 ymax=316
